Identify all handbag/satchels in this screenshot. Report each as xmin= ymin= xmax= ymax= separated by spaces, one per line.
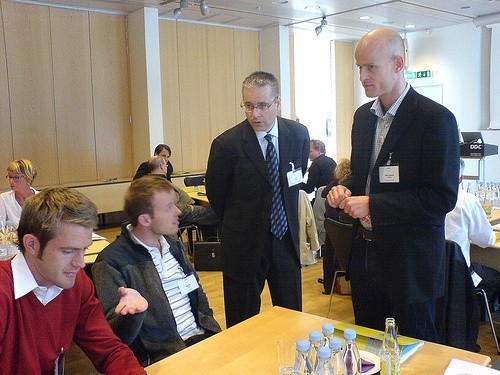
xmin=193 ymin=236 xmax=222 ymax=272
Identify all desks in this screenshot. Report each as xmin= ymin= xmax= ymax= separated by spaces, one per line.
xmin=83 ymin=232 xmax=110 ymax=264
xmin=143 ymin=306 xmax=493 ymax=375
xmin=185 ymin=189 xmax=212 ymax=256
xmin=469 ymin=202 xmax=500 ymax=271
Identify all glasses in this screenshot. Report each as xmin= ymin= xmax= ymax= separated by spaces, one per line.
xmin=162 ymin=163 xmax=168 ymax=167
xmin=240 ymin=98 xmax=277 ymax=112
xmin=6 ymin=174 xmax=23 ymax=180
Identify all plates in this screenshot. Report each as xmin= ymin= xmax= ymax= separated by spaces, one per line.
xmin=356 ymin=349 xmax=381 ymax=375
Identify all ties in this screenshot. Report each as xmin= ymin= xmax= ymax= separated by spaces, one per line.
xmin=264 ymin=134 xmax=288 ymax=240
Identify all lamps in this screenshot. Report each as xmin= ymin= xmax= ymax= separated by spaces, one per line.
xmin=315 ymin=17 xmax=328 ymax=36
xmin=173 ymin=0 xmax=188 ymax=21
xmin=200 ymin=0 xmax=209 ymax=16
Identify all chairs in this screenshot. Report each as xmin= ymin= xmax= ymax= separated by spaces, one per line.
xmin=311 ymin=186 xmax=327 ymax=259
xmin=178 ymin=223 xmax=202 ymax=258
xmin=444 ymin=239 xmax=500 ymax=356
xmin=54 ymin=261 xmax=99 ymax=375
xmin=298 ymin=190 xmax=322 ymax=267
xmin=323 ymin=218 xmax=353 ymax=317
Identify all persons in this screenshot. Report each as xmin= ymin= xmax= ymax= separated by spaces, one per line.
xmin=0 ymin=186 xmax=148 ymax=375
xmin=90 ymin=177 xmax=223 ymax=368
xmin=148 ymin=156 xmax=219 ymax=241
xmin=132 ymin=144 xmax=173 ymax=184
xmin=0 ymin=158 xmax=43 ymax=229
xmin=325 ymin=28 xmax=460 ymax=342
xmin=445 ymin=156 xmax=500 ymax=318
xmin=295 ymin=139 xmax=353 ymax=294
xmin=206 ymin=72 xmax=310 ymax=330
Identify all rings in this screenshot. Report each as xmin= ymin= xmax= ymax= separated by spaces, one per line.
xmin=330 ymin=197 xmax=335 ymax=199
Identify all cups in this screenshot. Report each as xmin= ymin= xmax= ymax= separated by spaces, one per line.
xmin=277 ymin=339 xmax=296 ymax=375
xmin=481 ymin=200 xmax=492 ymax=218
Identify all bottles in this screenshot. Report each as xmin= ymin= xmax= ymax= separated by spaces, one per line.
xmin=379 ymin=318 xmax=401 ymax=375
xmin=313 ymin=347 xmax=333 ymax=375
xmin=343 ymin=328 xmax=362 ymax=375
xmin=308 ymin=331 xmax=325 ymax=370
xmin=292 ymin=339 xmax=315 ymax=375
xmin=328 ymin=338 xmax=344 ymax=375
xmin=0 ymin=219 xmax=22 ymax=261
xmin=321 ymin=324 xmax=343 ymax=353
xmin=464 ymin=179 xmax=500 ymax=210
